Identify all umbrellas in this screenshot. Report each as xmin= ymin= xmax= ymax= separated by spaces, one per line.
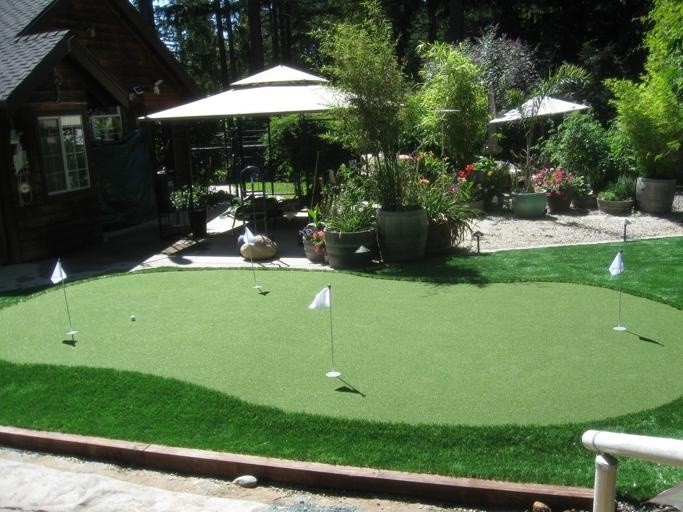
xmin=137 ymin=64 xmax=405 ymax=197
xmin=489 ymin=95 xmax=591 ymax=171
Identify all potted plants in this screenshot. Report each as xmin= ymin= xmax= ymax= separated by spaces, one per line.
xmin=168 ymin=182 xmax=210 ymax=240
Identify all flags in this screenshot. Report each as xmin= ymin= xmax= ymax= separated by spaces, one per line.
xmin=306 ymin=285 xmax=331 ymax=310
xmin=608 ymin=254 xmax=626 ymax=280
xmin=50 ymin=260 xmax=66 ymax=286
